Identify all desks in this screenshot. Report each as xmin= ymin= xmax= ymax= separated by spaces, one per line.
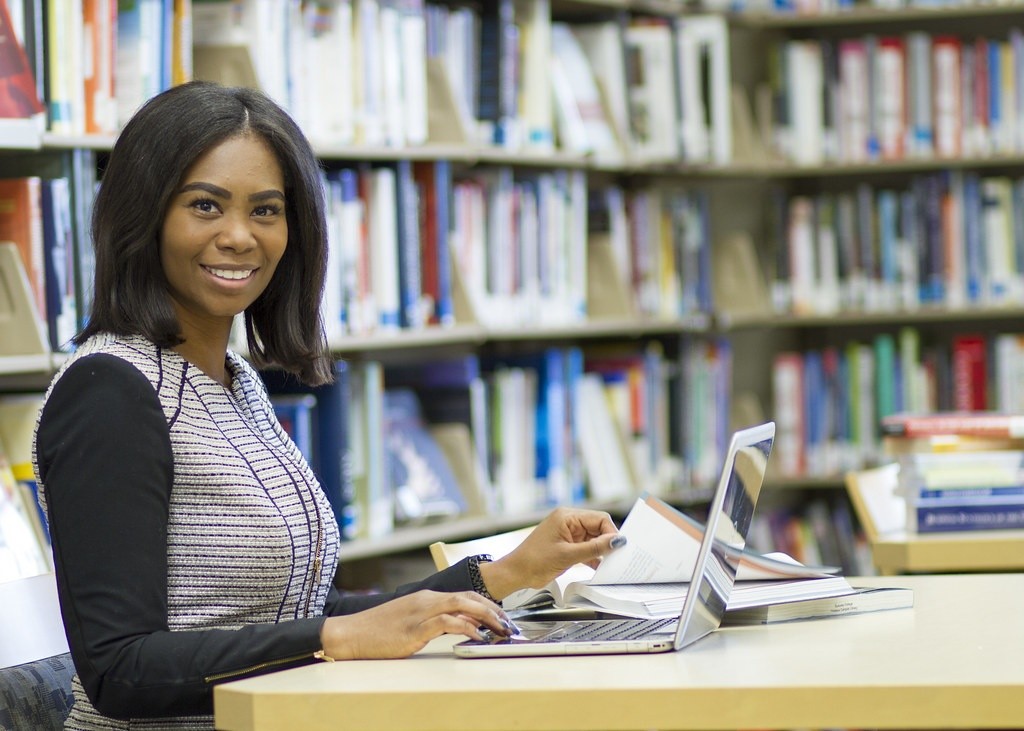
xmin=212 ymin=580 xmax=1024 ymax=730
xmin=842 ymin=474 xmax=1024 ymax=574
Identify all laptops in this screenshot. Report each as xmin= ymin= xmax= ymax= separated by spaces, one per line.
xmin=452 ymin=422 xmax=776 ymax=658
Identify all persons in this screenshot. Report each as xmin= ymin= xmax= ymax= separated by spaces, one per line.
xmin=32 ymin=80 xmax=627 ymax=730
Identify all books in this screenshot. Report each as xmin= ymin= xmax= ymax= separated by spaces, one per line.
xmin=0 ymin=324 xmax=1024 ymax=581
xmin=0 ymin=151 xmax=1024 ymax=359
xmin=1 ymin=0 xmax=1024 ymax=173
xmin=500 ymin=494 xmax=939 ymax=627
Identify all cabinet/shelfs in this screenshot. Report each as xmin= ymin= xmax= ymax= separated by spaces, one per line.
xmin=1 ymin=0 xmax=1024 ymax=576
